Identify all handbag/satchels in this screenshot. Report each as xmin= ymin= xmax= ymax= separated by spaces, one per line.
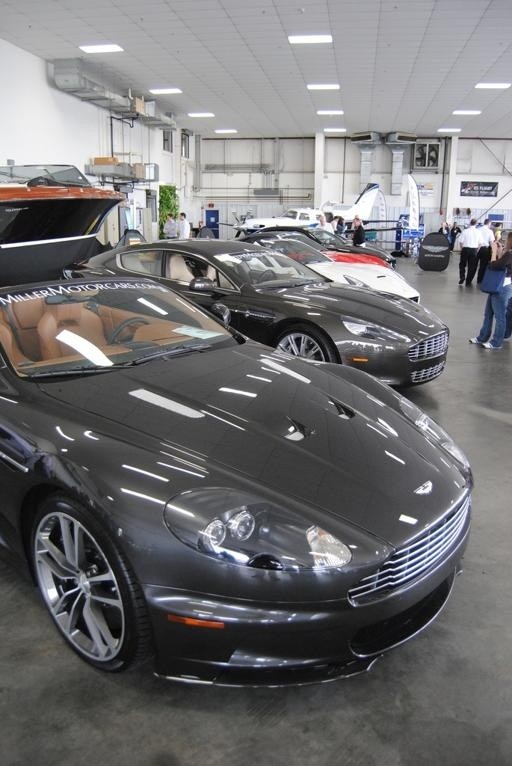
xmin=480 ymin=264 xmax=507 ymax=295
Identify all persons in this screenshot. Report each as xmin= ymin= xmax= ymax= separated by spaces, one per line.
xmin=177 ymin=212 xmax=191 ymax=240
xmin=459 ymin=218 xmax=481 ymax=287
xmin=316 ymin=212 xmax=366 ymax=248
xmin=468 ymin=231 xmax=511 ymax=350
xmin=450 ymin=221 xmax=462 ymax=252
xmin=438 ymin=222 xmax=449 ymax=238
xmin=494 ymin=221 xmax=502 ymax=248
xmin=163 ymin=212 xmax=177 ymax=238
xmin=477 ymin=218 xmax=496 ymax=287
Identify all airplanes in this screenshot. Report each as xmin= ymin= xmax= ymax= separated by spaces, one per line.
xmin=216 ymin=183 xmax=407 ymax=243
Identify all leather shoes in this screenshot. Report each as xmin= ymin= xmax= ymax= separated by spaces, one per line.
xmin=459 ymin=276 xmax=465 ymax=284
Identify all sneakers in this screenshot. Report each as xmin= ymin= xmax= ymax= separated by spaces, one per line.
xmin=469 ymin=337 xmax=484 ymax=344
xmin=482 ymin=342 xmax=503 ymax=349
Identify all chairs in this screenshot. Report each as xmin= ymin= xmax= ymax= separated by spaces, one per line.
xmin=0 ymin=293 xmax=106 ymax=367
xmin=122 ymin=253 xmax=249 ymax=289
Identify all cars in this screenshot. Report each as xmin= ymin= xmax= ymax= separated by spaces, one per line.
xmin=1 ymin=163 xmax=127 ymax=288
xmin=0 ymin=273 xmax=473 ymax=689
xmin=215 ymin=206 xmax=423 ymax=306
xmin=62 ymin=238 xmax=451 ymax=391
